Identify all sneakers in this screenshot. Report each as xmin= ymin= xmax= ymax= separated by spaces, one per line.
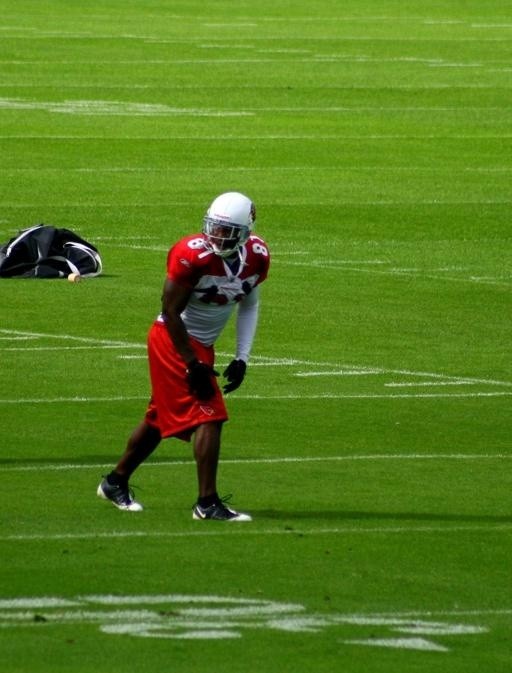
xmin=192 ymin=503 xmax=252 ymax=522
xmin=96 ymin=476 xmax=143 ymax=512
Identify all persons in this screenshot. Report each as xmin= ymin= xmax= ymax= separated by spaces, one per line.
xmin=95 ymin=192 xmax=271 ymax=523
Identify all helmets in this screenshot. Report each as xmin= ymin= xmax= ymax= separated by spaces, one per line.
xmin=200 ymin=192 xmax=255 ymax=258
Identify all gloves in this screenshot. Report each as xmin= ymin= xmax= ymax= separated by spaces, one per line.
xmin=222 ymin=359 xmax=246 ymax=394
xmin=186 ymin=360 xmax=219 ymax=401
